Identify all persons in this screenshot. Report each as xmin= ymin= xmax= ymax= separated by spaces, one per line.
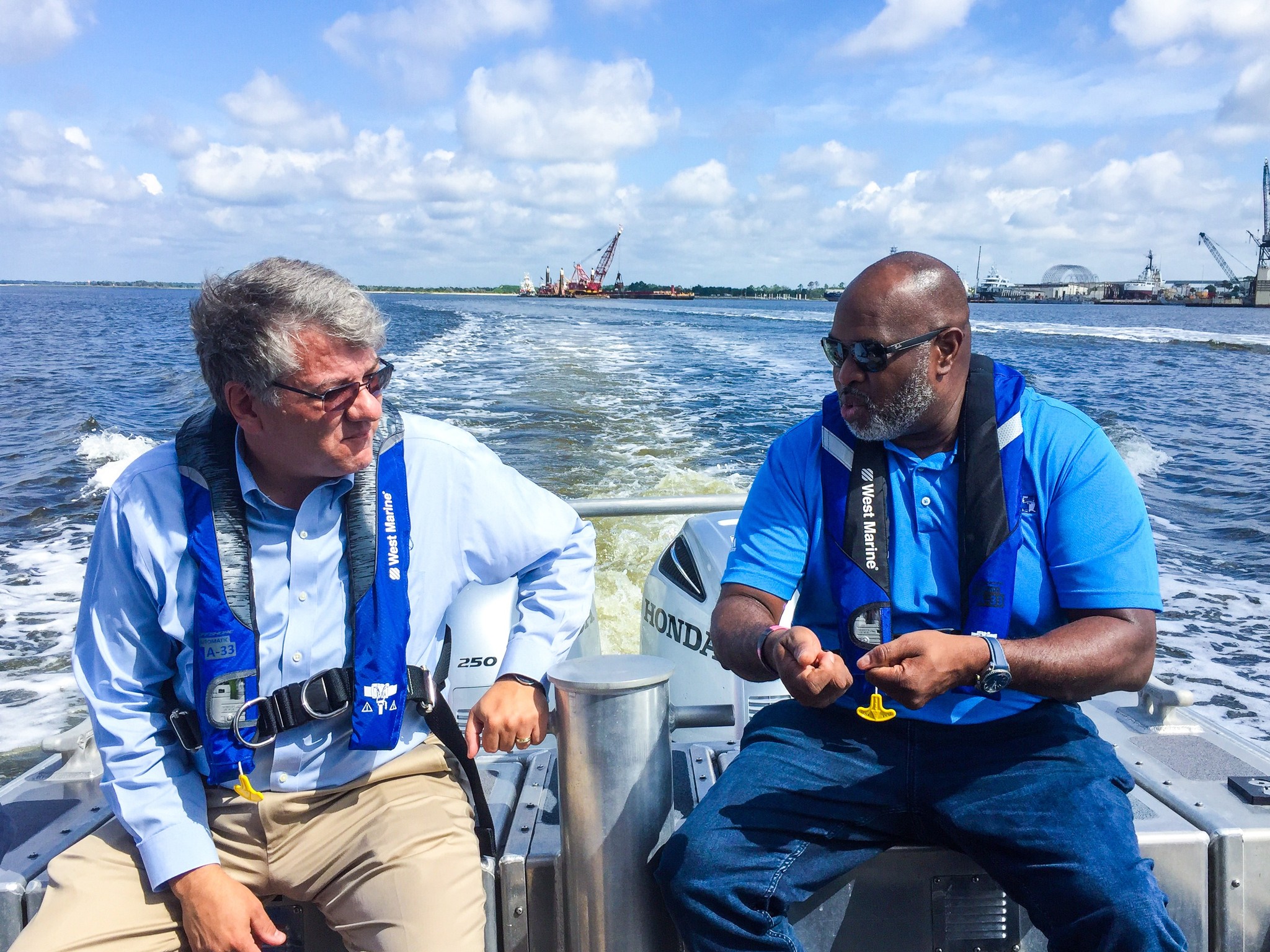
xmin=646 ymin=251 xmax=1188 ymax=952
xmin=4 ymin=258 xmax=597 ymax=952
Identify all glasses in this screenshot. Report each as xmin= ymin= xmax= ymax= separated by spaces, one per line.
xmin=265 ymin=355 xmax=394 ymax=414
xmin=820 ymin=327 xmax=965 ymax=373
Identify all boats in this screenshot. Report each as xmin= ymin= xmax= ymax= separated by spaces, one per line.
xmin=517 ymin=223 xmax=696 ymax=300
xmin=977 ymin=261 xmax=1021 ymax=299
xmin=823 ymin=291 xmax=843 ymax=301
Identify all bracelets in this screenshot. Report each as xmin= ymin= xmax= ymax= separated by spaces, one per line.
xmin=757 ymin=625 xmax=789 ymax=676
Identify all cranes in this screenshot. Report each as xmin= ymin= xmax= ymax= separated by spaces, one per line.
xmin=1198 ymin=232 xmax=1257 ymax=288
xmin=1245 ymin=156 xmax=1270 ymax=269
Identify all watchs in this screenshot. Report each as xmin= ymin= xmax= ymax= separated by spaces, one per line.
xmin=975 ymin=635 xmax=1013 ymax=693
xmin=495 ymin=673 xmax=547 ymax=699
